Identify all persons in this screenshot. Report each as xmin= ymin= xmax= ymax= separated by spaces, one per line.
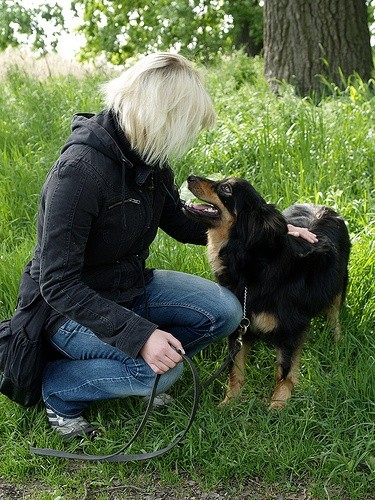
xmin=0 ymin=51 xmax=318 ymax=446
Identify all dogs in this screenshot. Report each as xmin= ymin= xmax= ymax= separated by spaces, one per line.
xmin=181 ymin=176 xmax=351 ymax=414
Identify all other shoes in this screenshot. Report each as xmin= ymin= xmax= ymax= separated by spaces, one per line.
xmin=46 ymin=405 xmax=96 ymax=440
xmin=143 ymin=392 xmax=174 ymax=409
